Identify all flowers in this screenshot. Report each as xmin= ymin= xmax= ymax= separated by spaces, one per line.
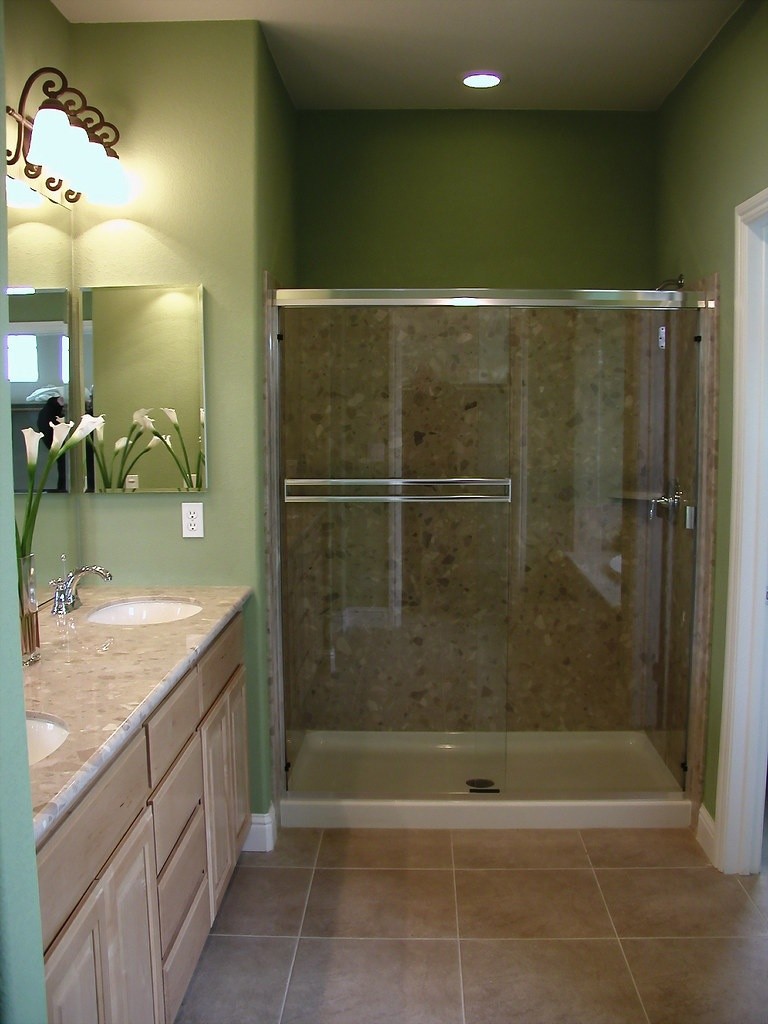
xmin=85 ymin=407 xmax=165 ymax=493
xmin=15 ymin=413 xmax=96 ymax=613
xmin=136 ymin=406 xmax=205 ymax=492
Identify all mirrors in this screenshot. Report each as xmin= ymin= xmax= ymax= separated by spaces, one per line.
xmin=77 ymin=284 xmax=210 ymax=492
xmin=7 ymin=172 xmax=81 ymax=605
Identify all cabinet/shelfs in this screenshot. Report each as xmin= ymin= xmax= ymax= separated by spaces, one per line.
xmin=35 ymin=610 xmax=252 ymax=1024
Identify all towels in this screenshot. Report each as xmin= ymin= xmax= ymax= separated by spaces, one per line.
xmin=24 ymin=383 xmax=92 ymax=405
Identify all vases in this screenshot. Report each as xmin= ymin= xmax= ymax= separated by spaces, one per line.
xmin=17 ymin=554 xmax=42 ymax=666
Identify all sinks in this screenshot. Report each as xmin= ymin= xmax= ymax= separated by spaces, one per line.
xmin=87 ymin=599 xmax=205 ymax=627
xmin=25 ymin=710 xmax=71 ymax=768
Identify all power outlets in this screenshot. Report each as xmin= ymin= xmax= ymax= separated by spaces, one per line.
xmin=182 ymin=502 xmax=205 ymax=538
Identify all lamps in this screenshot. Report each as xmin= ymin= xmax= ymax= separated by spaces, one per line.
xmin=7 ymin=60 xmax=135 ymax=212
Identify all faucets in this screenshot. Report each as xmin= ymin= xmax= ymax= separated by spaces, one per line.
xmin=647 ymin=496 xmax=673 ymax=521
xmin=63 ymin=564 xmax=113 ymax=604
